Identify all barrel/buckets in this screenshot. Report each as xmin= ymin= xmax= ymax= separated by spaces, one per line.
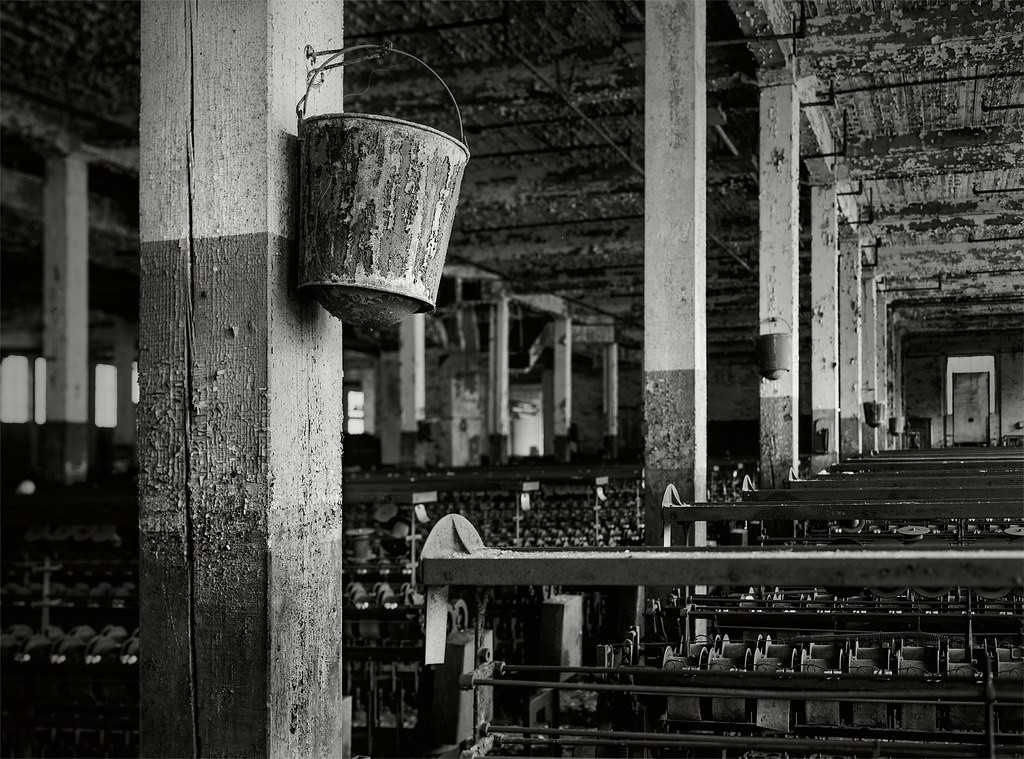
xmin=416 ymin=410 xmax=438 ymax=441
xmin=889 ymin=409 xmax=904 ymax=436
xmin=864 ymin=387 xmax=885 ymax=428
xmin=291 ymin=42 xmax=470 ymax=321
xmin=754 ymin=316 xmax=791 ymax=380
